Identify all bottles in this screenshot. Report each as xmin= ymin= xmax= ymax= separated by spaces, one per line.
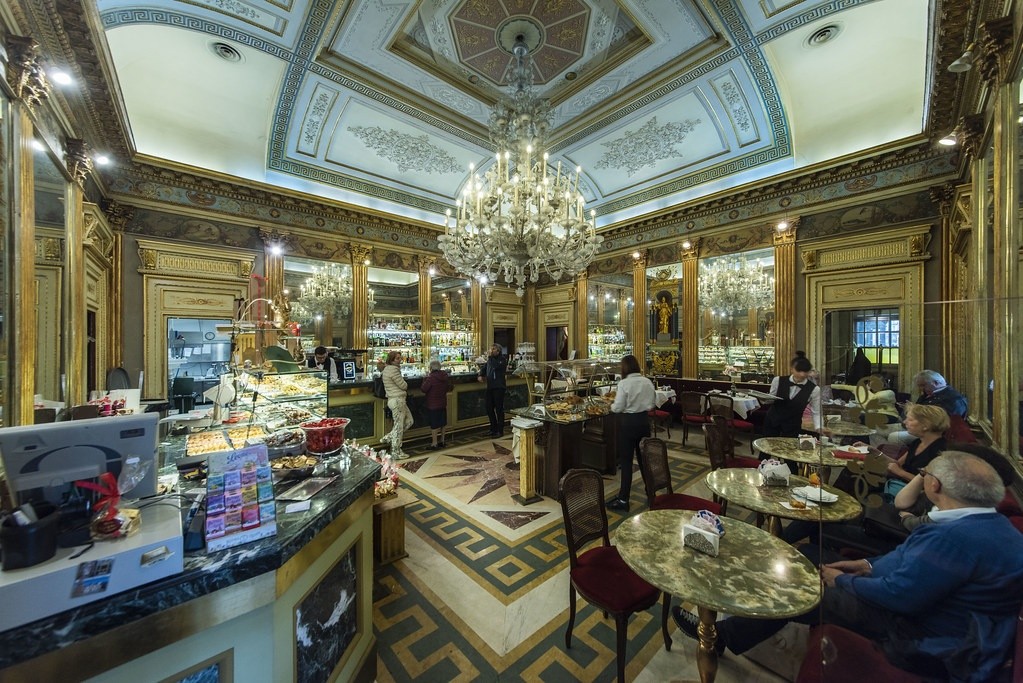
xmin=508 ymin=342 xmax=536 ymax=370
xmin=367 ymin=318 xmax=475 ymax=363
xmin=653 ymin=376 xmax=658 ymax=390
xmin=731 ymin=380 xmax=736 ymax=397
xmin=698 ymin=345 xmax=775 ymax=367
xmin=589 ymin=326 xmax=631 ymax=357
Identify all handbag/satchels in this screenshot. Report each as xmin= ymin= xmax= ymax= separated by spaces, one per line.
xmin=373 ymin=374 xmax=388 ymax=399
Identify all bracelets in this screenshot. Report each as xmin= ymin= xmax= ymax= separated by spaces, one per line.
xmin=875 ymin=452 xmax=883 ymax=459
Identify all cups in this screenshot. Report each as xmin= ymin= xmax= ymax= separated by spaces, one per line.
xmin=807 ymin=463 xmax=824 ymax=486
xmin=789 ymin=489 xmax=806 ymax=509
xmin=663 ymin=386 xmax=671 ymax=391
xmin=820 ymin=436 xmax=829 ymax=444
xmin=727 ymin=390 xmax=731 ymax=397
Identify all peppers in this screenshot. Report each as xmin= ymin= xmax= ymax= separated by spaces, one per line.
xmin=302 ymin=418 xmax=344 ymax=453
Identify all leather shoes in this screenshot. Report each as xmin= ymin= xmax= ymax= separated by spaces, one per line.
xmin=483 ymin=431 xmax=497 ymax=436
xmin=605 ymin=498 xmax=630 ymax=511
xmin=497 ymin=431 xmax=502 ymax=436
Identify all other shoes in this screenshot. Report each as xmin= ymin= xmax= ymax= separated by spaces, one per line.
xmin=380 ymin=438 xmax=387 ymax=444
xmin=393 ymin=453 xmax=410 ymax=460
xmin=426 ymin=444 xmax=439 ymax=450
xmin=438 ymin=441 xmax=446 ymax=447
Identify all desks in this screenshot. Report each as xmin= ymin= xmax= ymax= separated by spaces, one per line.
xmin=700 ymin=393 xmax=760 ymax=419
xmin=655 ymin=389 xmax=676 ymax=408
xmin=801 ymin=417 xmax=877 ymax=435
xmin=753 ymin=437 xmax=864 ymax=484
xmin=373 ymin=486 xmax=420 ymax=566
xmin=704 ymin=468 xmax=863 ymax=538
xmin=615 ymin=509 xmax=825 ymax=682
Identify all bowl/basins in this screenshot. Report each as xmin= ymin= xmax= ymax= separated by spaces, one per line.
xmin=271 ymin=464 xmax=314 ymax=477
xmin=299 ymin=417 xmax=351 ymax=452
xmin=267 ymin=441 xmax=307 ymax=459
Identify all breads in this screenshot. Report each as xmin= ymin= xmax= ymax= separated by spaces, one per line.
xmin=809 ymin=473 xmax=821 ymax=485
xmin=547 ymin=392 xmax=618 ymax=422
xmin=847 ymin=447 xmax=859 ymax=452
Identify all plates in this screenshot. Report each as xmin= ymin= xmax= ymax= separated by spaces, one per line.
xmin=792 ymin=487 xmax=838 ymax=502
xmin=307 ymin=445 xmax=343 ymax=456
xmin=836 ymin=446 xmax=869 ymax=454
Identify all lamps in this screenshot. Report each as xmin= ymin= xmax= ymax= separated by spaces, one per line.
xmin=939 ymin=126 xmax=962 ymax=145
xmin=697 ymin=252 xmax=775 ymax=321
xmin=948 ymin=38 xmax=983 ymax=72
xmin=437 ymin=34 xmax=605 ymax=298
xmin=296 ymin=261 xmax=376 ymax=324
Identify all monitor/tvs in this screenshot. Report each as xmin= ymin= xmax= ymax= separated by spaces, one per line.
xmin=0 ymin=411 xmax=160 ymax=514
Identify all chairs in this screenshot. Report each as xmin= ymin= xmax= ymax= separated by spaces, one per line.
xmin=558 ymin=390 xmax=1023 ymax=682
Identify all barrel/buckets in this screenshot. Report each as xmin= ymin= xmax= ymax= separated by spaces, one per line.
xmin=0 ymin=503 xmax=58 ymax=570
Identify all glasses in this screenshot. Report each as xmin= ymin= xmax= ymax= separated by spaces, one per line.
xmin=917 ymin=468 xmax=941 ymax=488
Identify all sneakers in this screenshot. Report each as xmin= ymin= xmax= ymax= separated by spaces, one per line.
xmin=672 ymin=606 xmax=724 ymax=656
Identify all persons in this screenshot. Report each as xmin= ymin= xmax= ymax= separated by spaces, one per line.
xmin=854 ymin=347 xmax=871 ymax=378
xmin=780 ymin=444 xmax=1013 ymax=567
xmin=652 ymin=296 xmax=672 ymax=334
xmin=380 ymin=351 xmax=414 ymax=460
xmin=809 ymin=371 xmax=833 ymax=404
xmin=306 ymin=346 xmax=338 ymax=380
xmin=421 ymin=361 xmax=454 ymax=450
xmin=870 ymin=370 xmax=967 ymax=445
xmin=478 ymin=344 xmax=507 ymax=436
xmin=758 ymin=351 xmax=832 ymax=473
xmin=606 ymin=355 xmax=655 ymax=511
xmin=859 ymin=375 xmax=899 ymax=423
xmin=671 ymin=452 xmax=1023 ymax=683
xmin=833 ymin=405 xmax=950 ymax=499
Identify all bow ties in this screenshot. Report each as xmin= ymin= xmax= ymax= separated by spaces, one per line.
xmin=317 ymin=362 xmax=325 ymax=365
xmin=789 ymin=381 xmax=803 ymax=388
